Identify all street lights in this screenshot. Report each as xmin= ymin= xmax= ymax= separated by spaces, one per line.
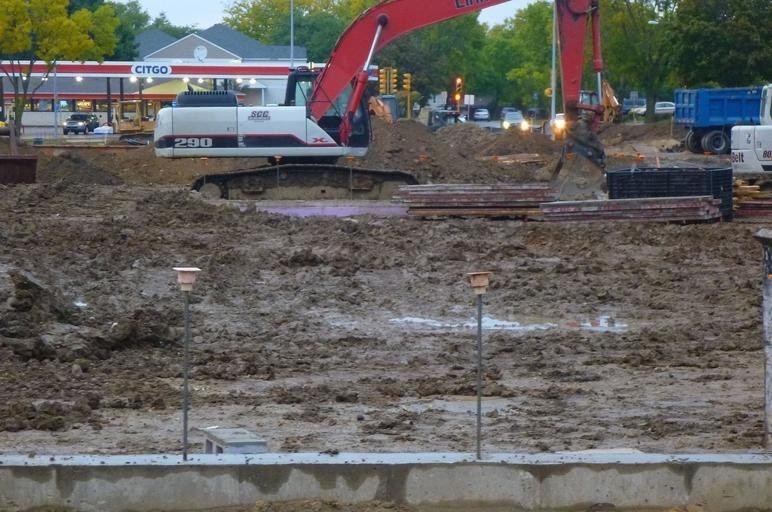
xmin=131 ymin=75 xmax=154 ymax=118
xmin=172 ymin=264 xmax=202 ymax=461
xmin=468 ymin=270 xmax=490 ymax=462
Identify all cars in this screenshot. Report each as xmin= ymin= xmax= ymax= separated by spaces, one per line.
xmin=500 ymin=106 xmax=516 ymax=120
xmin=503 ymin=112 xmax=527 ymax=131
xmin=473 ymin=109 xmax=489 ymax=121
xmin=634 ymin=101 xmax=674 ymax=117
xmin=525 ymin=109 xmax=547 ymax=120
xmin=64 ymin=113 xmax=100 ymax=134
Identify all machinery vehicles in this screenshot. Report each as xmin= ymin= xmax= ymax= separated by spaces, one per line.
xmin=728 ymin=85 xmax=772 ymax=216
xmin=365 ymin=90 xmax=405 ymax=124
xmin=114 ymin=98 xmax=161 ymax=146
xmin=153 ymin=0 xmax=620 ymax=203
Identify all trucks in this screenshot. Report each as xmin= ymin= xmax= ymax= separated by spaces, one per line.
xmin=672 ymin=84 xmax=772 ymax=153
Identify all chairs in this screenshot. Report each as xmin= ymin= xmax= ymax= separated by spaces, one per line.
xmin=307 ymin=87 xmax=312 ymax=96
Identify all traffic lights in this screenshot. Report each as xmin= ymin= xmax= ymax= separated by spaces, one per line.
xmin=454 ymin=93 xmax=460 ymax=100
xmin=403 ymin=72 xmax=411 ymax=90
xmin=379 ymin=66 xmax=389 ymax=94
xmin=389 ymin=67 xmax=398 ymax=94
xmin=456 ymin=78 xmax=462 ymax=91
